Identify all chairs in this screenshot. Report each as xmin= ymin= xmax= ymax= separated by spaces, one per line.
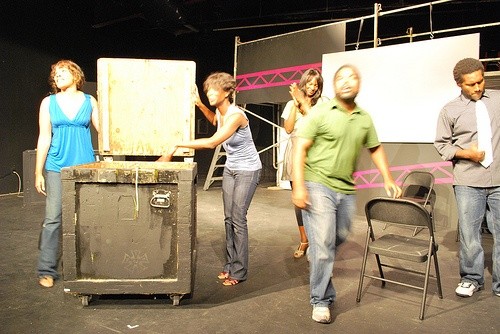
xmin=356 ymin=172 xmax=443 ymax=320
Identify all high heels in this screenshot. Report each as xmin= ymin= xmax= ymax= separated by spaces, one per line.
xmin=294 ymin=241 xmax=309 ymax=257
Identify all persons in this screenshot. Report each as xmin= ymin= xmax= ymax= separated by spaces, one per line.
xmin=432 ymin=58 xmax=500 ymax=298
xmin=278 ymin=68 xmax=330 ymax=258
xmin=34 ymin=60 xmax=98 ymax=288
xmin=156 ymin=73 xmax=263 ymax=285
xmin=292 ymin=66 xmax=403 ymax=324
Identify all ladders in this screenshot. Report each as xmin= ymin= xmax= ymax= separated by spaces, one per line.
xmin=203 ymin=104 xmax=254 ymax=190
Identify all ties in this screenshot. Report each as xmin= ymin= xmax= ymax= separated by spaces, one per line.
xmin=475 ymin=100 xmax=494 ymax=168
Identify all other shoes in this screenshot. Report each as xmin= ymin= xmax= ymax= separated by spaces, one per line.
xmin=222 ymin=278 xmax=238 ymax=286
xmin=40 ymin=275 xmax=52 ymax=287
xmin=218 ymin=271 xmax=229 ymax=279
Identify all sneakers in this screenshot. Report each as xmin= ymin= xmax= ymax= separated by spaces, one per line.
xmin=312 ymin=303 xmax=331 ymax=322
xmin=455 ymin=282 xmax=484 ymax=296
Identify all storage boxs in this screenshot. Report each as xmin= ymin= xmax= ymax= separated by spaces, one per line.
xmin=60 ymin=57 xmax=197 ymax=307
